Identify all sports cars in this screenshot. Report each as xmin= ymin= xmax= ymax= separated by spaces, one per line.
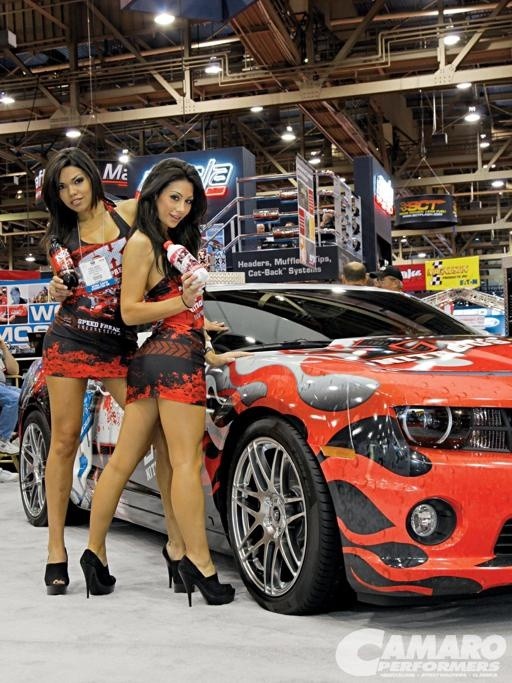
xmin=15 ymin=281 xmax=512 ymax=616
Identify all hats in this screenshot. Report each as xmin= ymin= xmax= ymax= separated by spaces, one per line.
xmin=370 ymin=268 xmax=402 ymax=281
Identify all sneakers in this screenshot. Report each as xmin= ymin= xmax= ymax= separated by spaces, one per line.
xmin=0 ymin=439 xmax=20 ymax=454
xmin=0 ymin=468 xmax=20 ymax=482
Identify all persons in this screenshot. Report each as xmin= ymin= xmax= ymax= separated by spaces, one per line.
xmin=257 ymin=208 xmax=334 ymax=249
xmin=80 ymin=157 xmax=254 ymax=607
xmin=342 ymin=262 xmax=369 ymax=285
xmin=10 ymin=287 xmax=26 ymax=304
xmin=45 ymin=147 xmax=142 ymax=586
xmin=382 ymin=267 xmax=403 ymax=291
xmin=0 ymin=337 xmax=23 ymax=455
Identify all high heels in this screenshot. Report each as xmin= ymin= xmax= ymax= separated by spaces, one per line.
xmin=45 ymin=548 xmax=70 ymax=595
xmin=79 ymin=550 xmax=116 ymax=598
xmin=162 ymin=546 xmax=235 ymax=607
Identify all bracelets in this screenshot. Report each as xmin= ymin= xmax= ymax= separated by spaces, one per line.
xmin=181 ymin=296 xmax=193 ymax=309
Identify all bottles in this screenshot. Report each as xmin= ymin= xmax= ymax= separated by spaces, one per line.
xmin=47 ymin=236 xmax=79 ymax=290
xmin=163 ymin=238 xmax=209 ymax=284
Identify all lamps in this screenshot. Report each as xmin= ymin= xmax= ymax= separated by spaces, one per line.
xmin=442 ymin=19 xmax=505 ymax=189
xmin=153 ymin=9 xmax=322 ymax=166
xmin=64 ymin=126 xmax=82 ymax=139
xmin=24 ymin=252 xmax=36 ymax=262
xmin=0 ymin=91 xmax=16 ymax=106
xmin=118 ymin=148 xmax=132 ymax=166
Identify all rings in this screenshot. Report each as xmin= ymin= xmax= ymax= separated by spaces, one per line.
xmin=51 ymin=296 xmax=56 ymax=301
